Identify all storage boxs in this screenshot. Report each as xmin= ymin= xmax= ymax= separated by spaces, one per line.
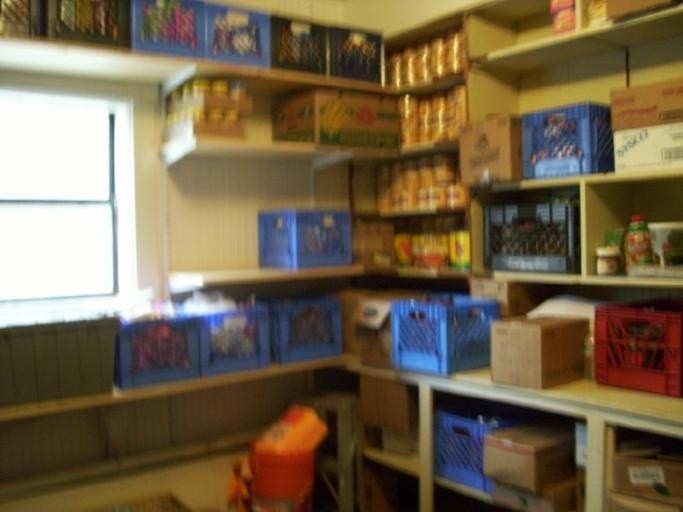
xmin=263 ymin=299 xmax=345 ymax=366
xmin=391 ymin=292 xmax=503 ymax=380
xmin=610 ymin=432 xmax=683 ymax=506
xmin=359 ymin=375 xmax=418 ymax=430
xmin=175 ymin=303 xmax=272 ymax=376
xmin=359 ymin=301 xmax=396 ymax=370
xmin=0 ymin=0 xmax=46 ymax=39
xmin=609 ymin=77 xmax=682 ymax=131
xmin=596 ymin=295 xmax=683 ymax=400
xmin=109 ymin=316 xmax=201 ymax=392
xmin=484 ymin=424 xmax=576 ymax=494
xmin=209 ymin=4 xmax=272 ymax=67
xmin=462 ymin=112 xmax=522 ymax=187
xmin=45 ymin=1 xmax=131 ymax=51
xmin=272 ymin=87 xmax=400 ymax=148
xmin=521 ymin=101 xmax=614 ymax=178
xmin=270 ymin=14 xmax=330 ymax=75
xmin=435 ymin=403 xmax=539 ymax=494
xmin=490 ymin=316 xmax=590 ymax=390
xmin=330 ymin=25 xmax=383 ymax=84
xmin=258 ymin=209 xmax=355 ymax=267
xmin=614 ymin=123 xmax=683 ymax=173
xmin=133 ymin=0 xmax=206 ymax=62
xmin=0 ymin=316 xmax=118 ymax=407
xmin=494 ymin=475 xmax=576 ymax=512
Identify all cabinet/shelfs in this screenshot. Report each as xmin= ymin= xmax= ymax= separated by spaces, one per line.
xmin=0 ymin=348 xmax=354 ymax=512
xmin=0 ymin=0 xmax=390 ymax=93
xmin=158 ymin=62 xmax=401 ymax=291
xmin=350 ymin=0 xmax=683 ymax=512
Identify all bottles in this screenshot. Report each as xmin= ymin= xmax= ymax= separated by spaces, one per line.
xmin=624 ymin=216 xmax=653 ymax=276
xmin=165 ymin=74 xmax=246 ymax=139
xmin=395 ymin=232 xmax=415 ymax=268
xmin=393 ymin=165 xmax=406 ymax=193
xmin=404 ymin=161 xmax=420 ymax=189
xmin=596 ymin=247 xmax=619 ymax=277
xmin=399 ymin=86 xmax=466 ymax=146
xmin=412 ymin=232 xmax=449 ymax=269
xmin=375 ymin=190 xmax=443 ymax=215
xmin=403 ymin=48 xmax=417 ymax=87
xmin=416 ymin=43 xmax=432 ymax=83
xmin=432 ymin=151 xmax=456 ymax=181
xmin=387 ymin=50 xmax=400 ymax=87
xmin=376 ymin=162 xmax=391 ymax=193
xmin=430 ymin=37 xmax=448 ymax=80
xmin=446 ymin=184 xmax=465 ymax=207
xmin=449 ymin=219 xmax=470 ymax=271
xmin=444 ymin=31 xmax=462 ymax=74
xmin=418 ymin=157 xmax=435 ymax=188
